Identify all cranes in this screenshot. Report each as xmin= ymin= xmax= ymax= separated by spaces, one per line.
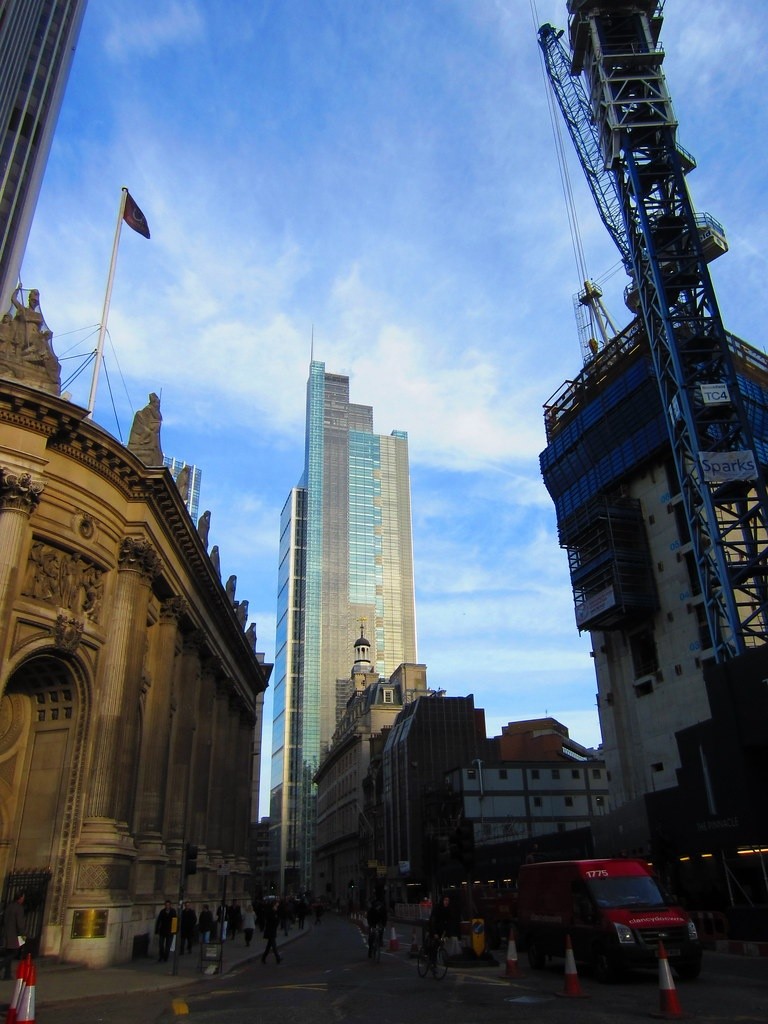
xmin=532 ymin=1 xmax=768 ymax=737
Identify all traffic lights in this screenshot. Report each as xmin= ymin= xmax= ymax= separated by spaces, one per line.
xmin=185 ymin=840 xmax=198 ymax=876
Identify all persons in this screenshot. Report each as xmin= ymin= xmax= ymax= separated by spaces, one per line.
xmin=177 ymin=463 xmax=256 ymax=647
xmin=155 ymin=900 xmax=176 ymax=962
xmin=130 ymin=393 xmax=162 ymax=451
xmin=261 ymin=900 xmax=284 ymax=964
xmin=199 ymin=904 xmax=214 ymax=944
xmin=216 ymin=895 xmax=325 ymax=947
xmin=424 ymin=896 xmax=452 ymax=972
xmin=180 ymin=901 xmax=197 ymax=955
xmin=0 ymin=283 xmax=53 ymax=354
xmin=0 ymin=890 xmax=25 ymax=980
xmin=367 ymin=900 xmax=387 ymax=958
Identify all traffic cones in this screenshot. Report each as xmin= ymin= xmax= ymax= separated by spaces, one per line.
xmin=388 ymin=926 xmax=401 ymax=951
xmin=348 ymin=911 xmax=370 ymax=935
xmin=554 ymin=934 xmax=593 ymax=999
xmin=497 ymin=928 xmax=527 ymax=979
xmin=7 ymin=953 xmax=42 ymax=1024
xmin=650 ymin=940 xmax=698 ymax=1019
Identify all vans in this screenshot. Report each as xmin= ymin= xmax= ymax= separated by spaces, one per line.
xmin=512 ymin=859 xmax=705 ymax=983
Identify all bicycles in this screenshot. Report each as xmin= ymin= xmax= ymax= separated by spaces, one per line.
xmin=413 ymin=927 xmax=451 ymax=979
xmin=365 ymin=926 xmax=388 ymax=964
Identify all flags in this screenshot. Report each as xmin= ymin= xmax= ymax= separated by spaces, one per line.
xmin=123 ymin=193 xmax=150 ymax=239
